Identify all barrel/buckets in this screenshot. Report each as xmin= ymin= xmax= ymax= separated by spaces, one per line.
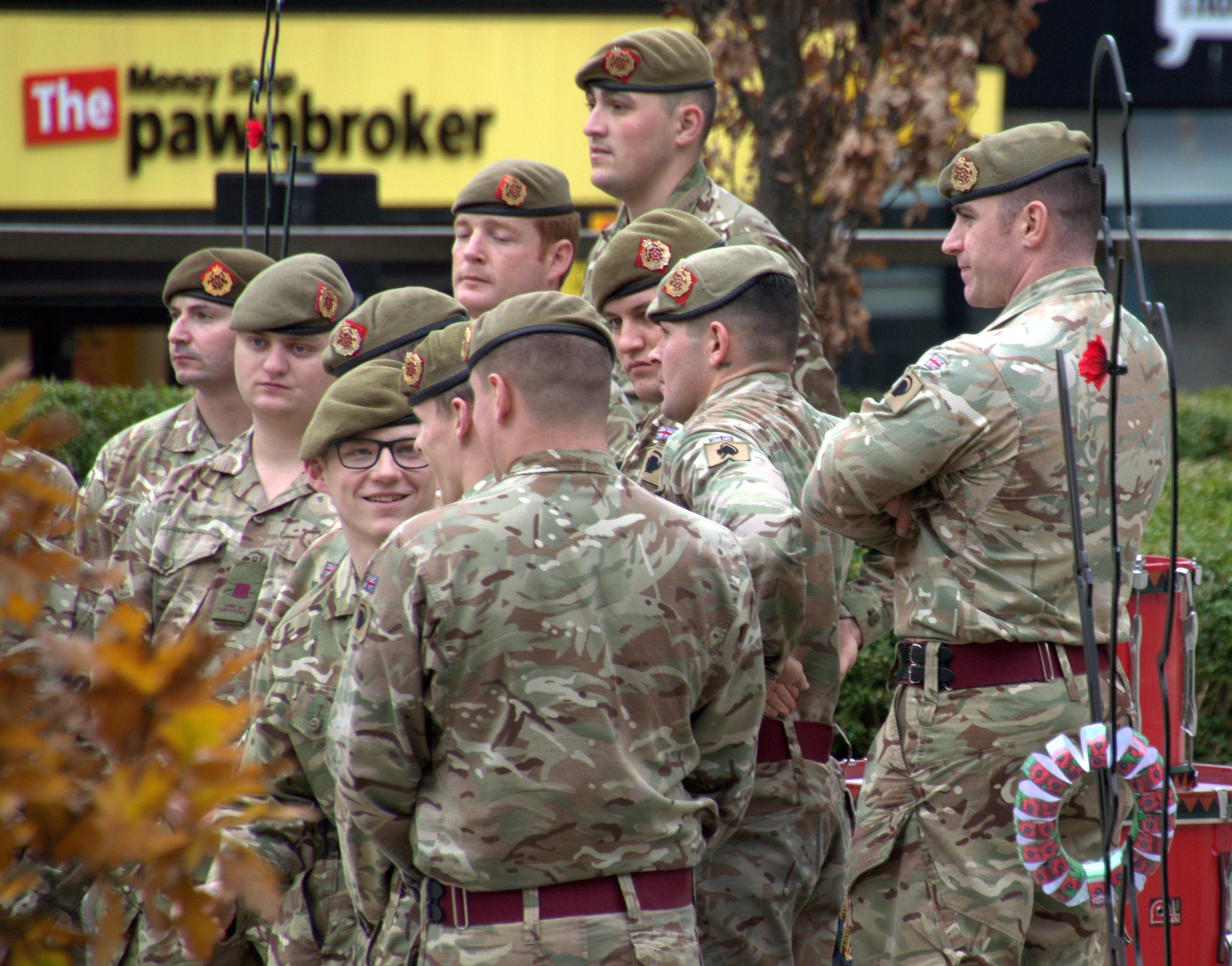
xmin=1114 ymin=555 xmax=1200 ymax=791
xmin=840 ymin=758 xmax=1231 ymax=965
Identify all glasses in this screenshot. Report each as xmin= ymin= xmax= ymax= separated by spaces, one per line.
xmin=330 ymin=437 xmax=429 ymax=469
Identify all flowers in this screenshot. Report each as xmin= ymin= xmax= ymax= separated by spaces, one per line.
xmin=1075 ymin=332 xmax=1109 ymax=393
xmin=244 ymin=116 xmax=268 ymax=150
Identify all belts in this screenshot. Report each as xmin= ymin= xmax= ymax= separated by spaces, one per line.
xmin=428 ymin=868 xmax=693 ymax=930
xmin=757 ymin=717 xmax=834 ymax=764
xmin=895 ymin=642 xmax=1109 ymax=692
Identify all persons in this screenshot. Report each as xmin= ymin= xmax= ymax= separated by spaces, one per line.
xmin=168 ymin=357 xmax=438 ymax=966
xmin=397 ymin=319 xmax=505 ymax=504
xmin=588 ymin=206 xmax=728 ymax=502
xmin=0 ymin=434 xmax=99 ymax=966
xmin=644 ymin=244 xmax=898 ymax=966
xmin=319 ymin=289 xmax=763 ymax=966
xmin=803 ymin=122 xmax=1175 ymax=966
xmin=572 ymin=25 xmax=849 ymax=421
xmin=77 ymin=243 xmax=274 ymax=966
xmin=93 ymin=254 xmax=362 ymax=966
xmin=450 ymin=158 xmax=638 ymax=466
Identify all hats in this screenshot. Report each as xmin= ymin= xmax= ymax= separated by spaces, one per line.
xmin=323 ymin=286 xmax=469 ymax=376
xmin=299 ymin=358 xmax=421 ymax=460
xmin=451 ymin=160 xmax=573 ymax=215
xmin=938 ymin=121 xmax=1092 ymax=205
xmin=646 ymin=245 xmax=797 ymax=327
xmin=461 ymin=290 xmax=617 ymax=370
xmin=592 ymin=209 xmax=725 ymax=313
xmin=228 ymin=252 xmax=356 ymax=335
xmin=163 ymin=247 xmax=276 ymax=309
xmin=400 ymin=320 xmax=469 ymax=407
xmin=575 ymin=29 xmax=717 ymax=91
